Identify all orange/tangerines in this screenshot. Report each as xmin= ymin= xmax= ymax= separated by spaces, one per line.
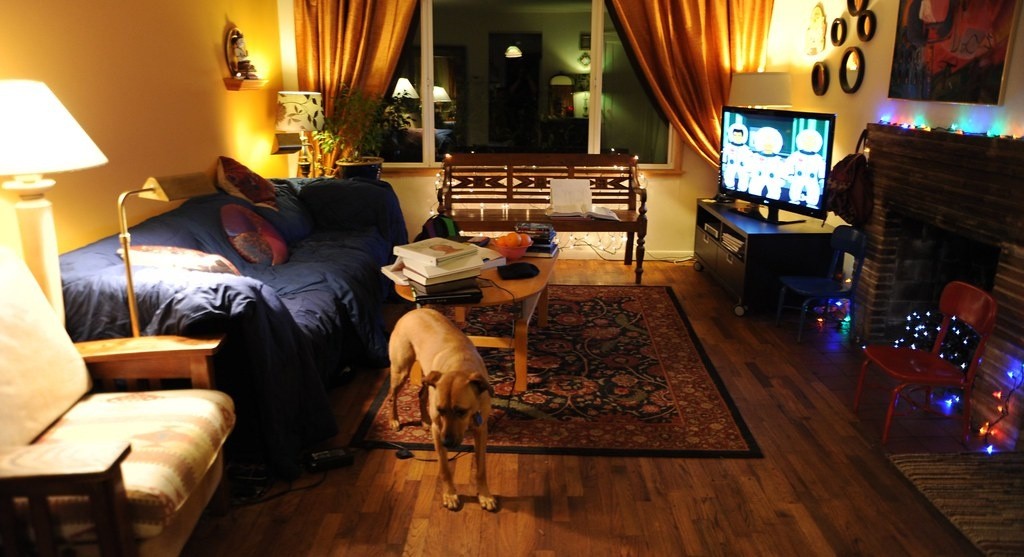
xmin=495 ymin=232 xmax=532 ymax=248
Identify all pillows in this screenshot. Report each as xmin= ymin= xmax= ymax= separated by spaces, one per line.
xmin=219 ymin=204 xmax=289 ymax=266
xmin=114 ymin=240 xmax=245 ymax=276
xmin=215 ymin=156 xmax=283 ymax=212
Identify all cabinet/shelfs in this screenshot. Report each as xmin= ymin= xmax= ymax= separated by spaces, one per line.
xmin=693 ymin=197 xmax=838 ymax=309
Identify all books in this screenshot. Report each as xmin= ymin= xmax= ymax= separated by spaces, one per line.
xmin=545 ymin=179 xmax=620 ymax=220
xmin=384 ymin=237 xmax=506 ymax=304
xmin=514 ymin=222 xmax=558 ymax=257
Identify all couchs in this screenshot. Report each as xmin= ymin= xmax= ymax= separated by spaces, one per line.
xmin=58 ymin=175 xmax=408 ymax=452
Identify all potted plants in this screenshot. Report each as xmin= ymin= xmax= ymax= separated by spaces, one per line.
xmin=311 ymin=77 xmax=423 ymax=181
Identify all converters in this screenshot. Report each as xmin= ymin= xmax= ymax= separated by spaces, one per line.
xmin=304 ymin=448 xmax=354 ymax=476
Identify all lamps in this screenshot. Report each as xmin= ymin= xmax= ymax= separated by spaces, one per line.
xmin=504 ymin=45 xmax=523 ymax=58
xmin=392 ymin=77 xmax=419 ymax=100
xmin=728 ymin=70 xmax=792 ymax=109
xmin=0 ymin=79 xmax=109 ymax=332
xmin=274 ymin=91 xmax=326 ymax=178
xmin=431 ymin=86 xmax=452 ymax=112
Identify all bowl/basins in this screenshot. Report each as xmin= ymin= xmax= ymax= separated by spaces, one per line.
xmin=490 ymin=240 xmax=533 ymax=260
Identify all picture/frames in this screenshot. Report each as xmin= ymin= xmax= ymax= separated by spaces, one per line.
xmin=581 ymin=35 xmax=590 ymax=50
xmin=885 ymin=0 xmax=1023 ymax=107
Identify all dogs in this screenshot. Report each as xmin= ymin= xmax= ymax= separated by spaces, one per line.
xmin=387 ymin=308 xmax=499 ymax=512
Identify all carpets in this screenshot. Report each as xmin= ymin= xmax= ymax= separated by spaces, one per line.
xmin=348 ymin=284 xmax=764 ymax=461
xmin=884 ymin=450 xmax=1024 ymax=557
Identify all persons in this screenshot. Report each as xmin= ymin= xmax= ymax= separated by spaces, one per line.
xmin=721 ymin=123 xmax=825 ymax=209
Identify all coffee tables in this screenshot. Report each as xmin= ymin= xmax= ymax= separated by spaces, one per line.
xmin=394 ymin=247 xmax=560 ymax=393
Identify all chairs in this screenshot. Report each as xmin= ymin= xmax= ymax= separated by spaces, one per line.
xmin=775 ymin=224 xmax=869 ymax=345
xmin=0 ymin=198 xmax=235 ymax=557
xmin=851 ymin=280 xmax=997 ymax=446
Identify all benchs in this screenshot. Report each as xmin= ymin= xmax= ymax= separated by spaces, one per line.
xmin=434 ymin=153 xmax=647 ymax=284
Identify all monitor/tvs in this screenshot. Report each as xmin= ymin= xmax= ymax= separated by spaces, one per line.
xmin=719 ymin=105 xmax=837 ymax=224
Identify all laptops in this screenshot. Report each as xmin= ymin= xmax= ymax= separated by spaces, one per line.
xmin=410 ymin=287 xmax=483 ymax=304
